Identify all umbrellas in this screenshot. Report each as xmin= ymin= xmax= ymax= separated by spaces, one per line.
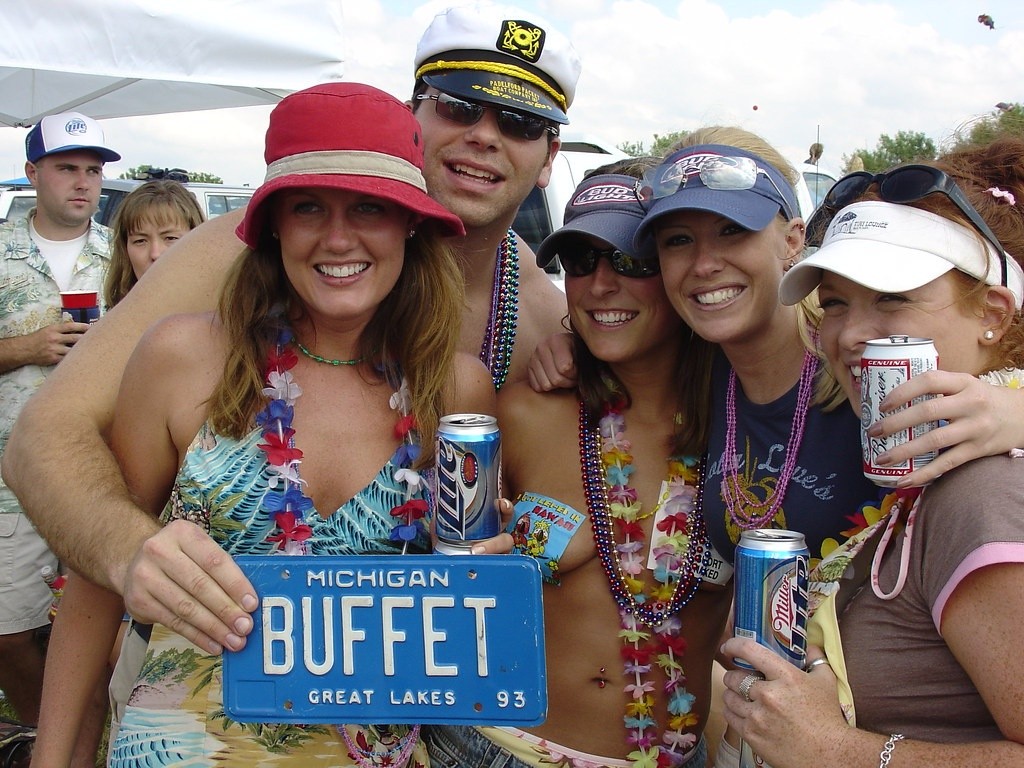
xmin=0 ymin=0 xmax=367 ymax=129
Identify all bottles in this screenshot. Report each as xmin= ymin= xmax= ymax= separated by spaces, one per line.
xmin=41 ymin=566 xmax=67 ymax=620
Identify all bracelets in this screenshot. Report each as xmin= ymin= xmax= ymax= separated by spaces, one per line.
xmin=880 ymin=734 xmax=904 ymax=768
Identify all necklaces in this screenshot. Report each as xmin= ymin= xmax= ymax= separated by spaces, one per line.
xmin=579 ymin=384 xmax=713 ymax=768
xmin=479 ymin=226 xmax=519 ymax=393
xmin=720 ymin=329 xmax=821 ymax=530
xmin=255 ymin=331 xmax=431 ymax=768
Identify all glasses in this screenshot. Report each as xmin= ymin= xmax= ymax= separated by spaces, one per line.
xmin=559 ymin=240 xmax=662 ymax=278
xmin=641 ymin=157 xmax=795 ymax=221
xmin=416 ymin=92 xmax=559 ymax=141
xmin=825 ymin=163 xmax=1007 ymax=286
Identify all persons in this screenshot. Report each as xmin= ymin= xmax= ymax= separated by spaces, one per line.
xmin=525 ymin=126 xmax=1024 ymax=768
xmin=722 ymin=143 xmax=1024 ymax=768
xmin=0 ymin=3 xmax=582 ymax=768
xmin=105 ymin=181 xmax=206 ymax=310
xmin=29 ymin=83 xmax=514 ymax=768
xmin=0 ymin=110 xmax=122 ymax=768
xmin=411 ymin=157 xmax=735 ymax=768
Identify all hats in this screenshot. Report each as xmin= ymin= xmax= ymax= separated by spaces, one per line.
xmin=412 ymin=5 xmax=582 ymax=125
xmin=633 ymin=144 xmax=805 ymax=254
xmin=235 ymin=82 xmax=467 ymax=249
xmin=25 ymin=112 xmax=121 ymax=164
xmin=778 ymin=201 xmax=1024 ymax=315
xmin=536 ymin=174 xmax=659 ymax=267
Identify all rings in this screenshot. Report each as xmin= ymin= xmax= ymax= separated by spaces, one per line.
xmin=806 ymin=658 xmax=829 ymax=672
xmin=739 ymin=675 xmax=764 ymax=701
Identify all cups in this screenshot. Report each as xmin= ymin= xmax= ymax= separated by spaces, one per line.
xmin=59 ymin=290 xmax=98 ymax=309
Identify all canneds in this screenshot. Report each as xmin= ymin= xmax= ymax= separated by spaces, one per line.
xmin=732 ymin=529 xmax=810 ymax=671
xmin=860 ymin=335 xmax=941 ymax=488
xmin=434 ymin=413 xmax=503 ymax=555
